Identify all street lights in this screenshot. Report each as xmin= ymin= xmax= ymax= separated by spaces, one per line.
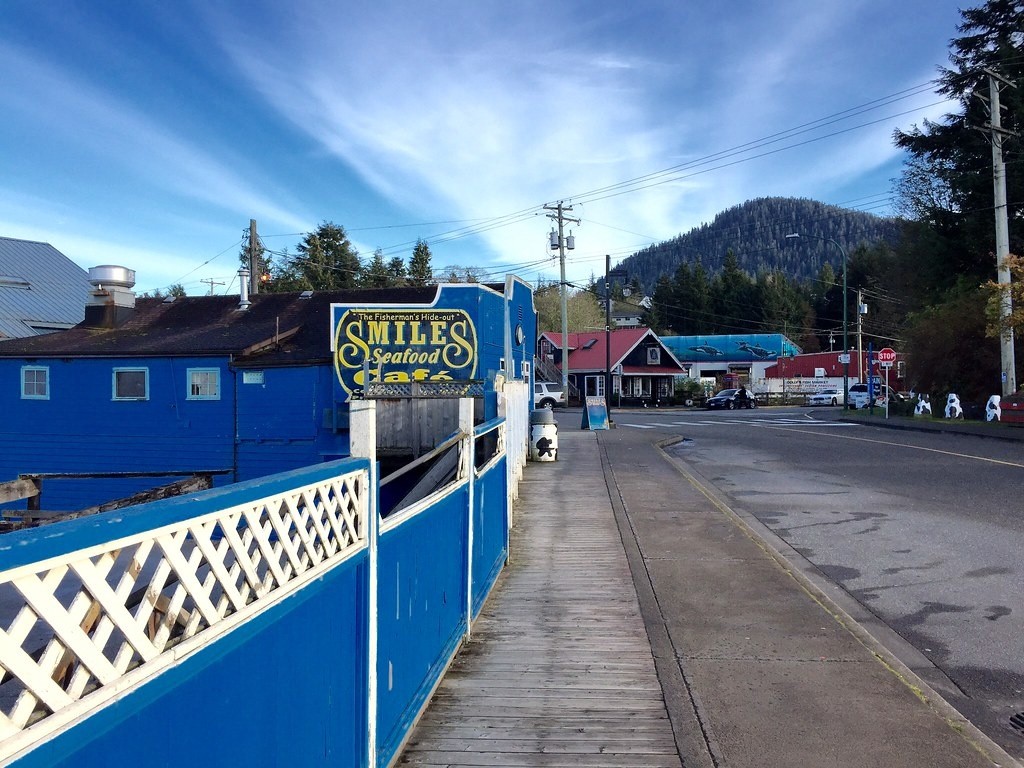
xmin=785 ymin=232 xmax=850 ymax=412
xmin=604 ymin=254 xmax=632 ymax=425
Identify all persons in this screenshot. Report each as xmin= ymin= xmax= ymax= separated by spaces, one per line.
xmin=737 ymin=383 xmax=748 ymax=409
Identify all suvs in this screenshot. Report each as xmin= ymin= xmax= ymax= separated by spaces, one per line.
xmin=846 ymin=382 xmax=907 ymax=411
xmin=535 ymin=380 xmax=565 ymax=411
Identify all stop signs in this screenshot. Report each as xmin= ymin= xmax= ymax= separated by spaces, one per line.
xmin=878 ymin=347 xmax=896 ymax=363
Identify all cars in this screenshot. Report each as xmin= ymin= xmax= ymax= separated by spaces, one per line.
xmin=997 ymin=391 xmax=1024 ymax=423
xmin=809 ymin=389 xmax=844 ymax=406
xmin=706 ymin=389 xmax=757 ymax=410
